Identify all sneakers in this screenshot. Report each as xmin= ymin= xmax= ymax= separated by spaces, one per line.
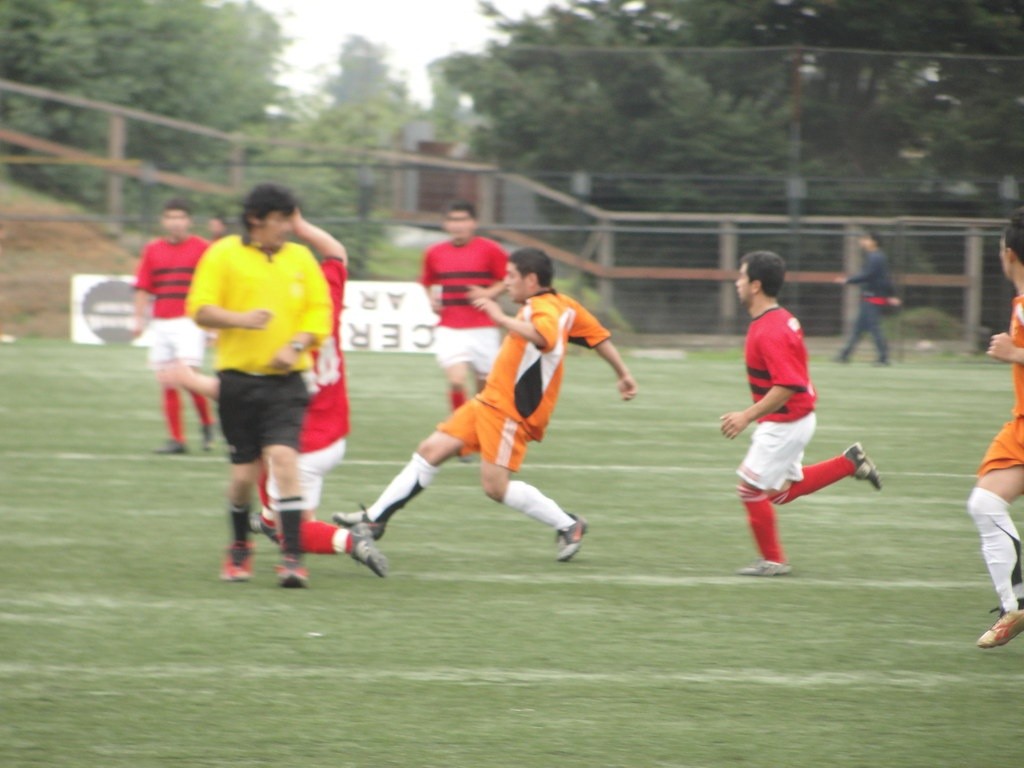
xmin=249 ymin=513 xmax=262 ymax=532
xmin=346 ymin=522 xmax=390 ymax=579
xmin=275 ymin=551 xmax=309 ymax=587
xmin=332 ymin=503 xmax=386 ymax=541
xmin=735 ymin=561 xmax=791 ymax=576
xmin=555 ymin=512 xmax=587 ymax=561
xmin=975 ymin=609 xmax=1024 ymax=648
xmin=221 ymin=541 xmax=252 ymax=579
xmin=843 ymin=443 xmax=881 ymax=490
xmin=989 ymin=597 xmax=1024 ymax=619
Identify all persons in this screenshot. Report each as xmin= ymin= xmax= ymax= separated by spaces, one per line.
xmin=719 ymin=251 xmax=883 ymax=578
xmin=187 ymin=184 xmax=388 ymax=587
xmin=966 ymin=204 xmax=1024 ymax=650
xmin=328 ymin=201 xmax=639 ymax=562
xmin=129 ymin=201 xmax=218 ymax=454
xmin=830 ymin=234 xmax=898 ymax=367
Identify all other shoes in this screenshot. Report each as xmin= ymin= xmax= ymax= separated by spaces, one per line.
xmin=151 ymin=438 xmax=188 ymax=455
xmin=202 ymin=422 xmax=219 ymax=451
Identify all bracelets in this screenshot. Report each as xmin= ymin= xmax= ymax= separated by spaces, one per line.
xmin=289 ymin=340 xmax=305 ymax=353
